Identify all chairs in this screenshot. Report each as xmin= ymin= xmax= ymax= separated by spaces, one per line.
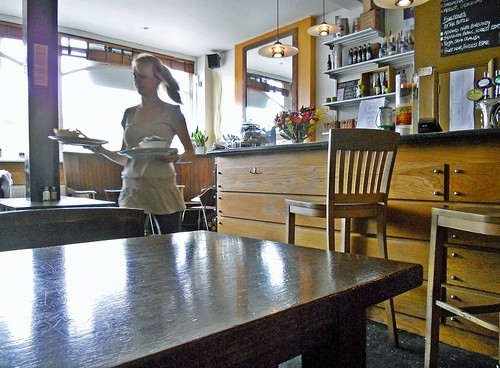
xmin=0 ymin=187 xmax=216 ymax=251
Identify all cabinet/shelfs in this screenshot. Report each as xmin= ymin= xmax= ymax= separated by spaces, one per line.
xmin=322 ymin=27 xmax=414 ymax=111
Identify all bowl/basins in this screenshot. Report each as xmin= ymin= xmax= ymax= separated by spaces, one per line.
xmin=139 ymin=140 xmax=166 ymax=149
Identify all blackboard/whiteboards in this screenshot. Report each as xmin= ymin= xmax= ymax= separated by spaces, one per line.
xmin=440 ymin=0 xmax=500 ymax=57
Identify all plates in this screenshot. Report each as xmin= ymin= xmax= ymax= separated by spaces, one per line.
xmin=48 ymin=136 xmax=109 ymax=149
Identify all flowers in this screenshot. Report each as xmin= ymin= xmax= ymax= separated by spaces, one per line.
xmin=274 ymin=107 xmax=329 ymax=142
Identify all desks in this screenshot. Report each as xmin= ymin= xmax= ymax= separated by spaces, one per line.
xmin=0 ymin=231 xmax=423 ymax=368
xmin=0 ymin=196 xmax=119 ymax=212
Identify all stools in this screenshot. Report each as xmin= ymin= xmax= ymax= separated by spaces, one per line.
xmin=286 ymin=128 xmax=401 ymax=350
xmin=424 ymin=206 xmax=500 ymax=368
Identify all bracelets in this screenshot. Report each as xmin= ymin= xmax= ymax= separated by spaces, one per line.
xmin=176 ymin=155 xmax=182 ymax=164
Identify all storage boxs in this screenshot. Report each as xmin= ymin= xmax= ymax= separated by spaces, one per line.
xmin=360 ymin=0 xmax=381 ymax=31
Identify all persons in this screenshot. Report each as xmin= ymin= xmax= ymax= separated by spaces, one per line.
xmin=83 ymin=55 xmax=195 ymax=235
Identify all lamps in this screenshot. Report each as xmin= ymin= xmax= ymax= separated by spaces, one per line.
xmin=257 ymin=0 xmax=299 ymax=58
xmin=373 ymin=0 xmax=431 ymax=9
xmin=307 ymin=0 xmax=341 ymax=37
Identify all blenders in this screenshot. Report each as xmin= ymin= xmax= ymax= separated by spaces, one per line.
xmin=375 ymin=105 xmax=397 ymax=132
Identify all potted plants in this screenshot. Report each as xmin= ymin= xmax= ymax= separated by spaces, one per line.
xmin=191 ymin=125 xmax=209 ymax=155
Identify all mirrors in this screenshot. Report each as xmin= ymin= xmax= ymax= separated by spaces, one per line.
xmin=242 ymin=27 xmax=298 ymax=132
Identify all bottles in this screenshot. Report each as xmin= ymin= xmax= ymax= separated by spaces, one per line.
xmin=375 ymin=74 xmax=381 ymax=95
xmin=349 ymin=48 xmax=353 ymax=65
xmin=362 ymin=44 xmax=366 ymax=62
xmin=51 ymin=186 xmax=58 ymax=200
xmin=366 ymin=43 xmax=371 ymax=61
xmin=327 ymin=54 xmax=331 ymax=70
xmin=353 ymin=47 xmax=357 ymax=64
xmin=357 ymin=46 xmax=362 ymax=63
xmin=43 ymin=185 xmax=51 ymax=201
xmin=382 ymin=72 xmax=388 ymax=94
